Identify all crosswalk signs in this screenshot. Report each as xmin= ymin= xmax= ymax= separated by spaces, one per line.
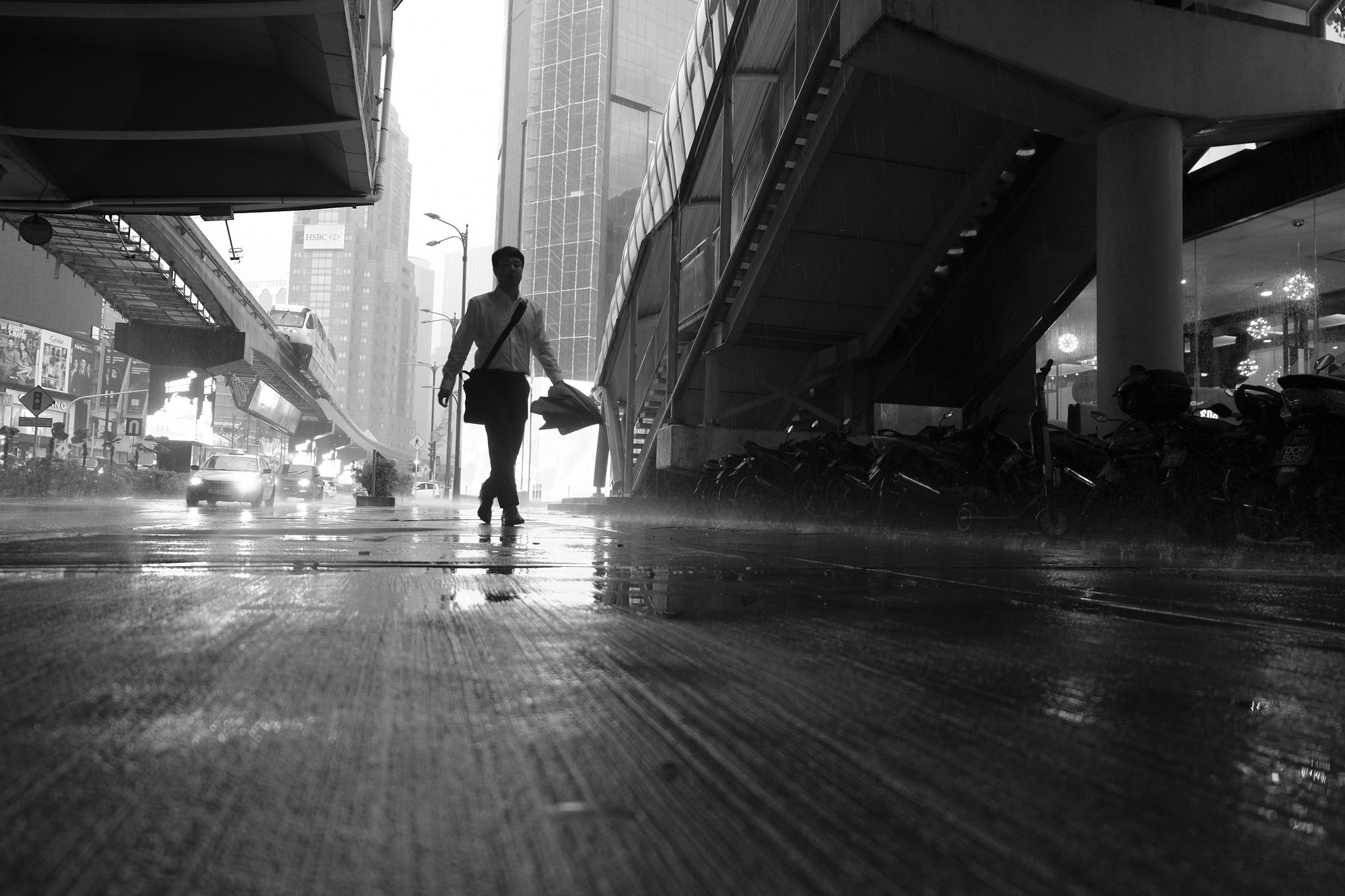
xmin=420 ymin=464 xmax=432 ymax=475
xmin=408 ymin=434 xmax=426 ymax=451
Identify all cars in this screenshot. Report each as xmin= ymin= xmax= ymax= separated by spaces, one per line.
xmin=352 ymin=486 xmax=370 ymax=500
xmin=186 ymin=448 xmax=278 ymax=510
xmin=272 ymin=464 xmax=324 ymax=503
xmin=321 ymin=480 xmax=337 ymax=501
xmin=411 ymin=482 xmax=440 ymax=501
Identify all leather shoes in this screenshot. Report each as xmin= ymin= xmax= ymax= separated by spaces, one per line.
xmin=501 ymin=507 xmax=525 ymax=526
xmin=478 ymin=499 xmax=492 ymax=524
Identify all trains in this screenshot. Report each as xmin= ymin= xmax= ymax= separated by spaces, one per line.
xmin=267 ymin=304 xmax=337 ymax=399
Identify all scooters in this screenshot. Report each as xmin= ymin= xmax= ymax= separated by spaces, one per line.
xmin=690 ymin=354 xmax=1345 ymax=546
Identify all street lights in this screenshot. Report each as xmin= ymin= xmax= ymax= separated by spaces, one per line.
xmin=424 ymin=212 xmax=469 ymax=503
xmin=399 ymin=361 xmax=446 ymax=481
xmin=419 ymin=307 xmax=462 ymax=501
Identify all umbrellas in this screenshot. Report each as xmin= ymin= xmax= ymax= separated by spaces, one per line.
xmin=529 ymin=381 xmax=605 ymax=435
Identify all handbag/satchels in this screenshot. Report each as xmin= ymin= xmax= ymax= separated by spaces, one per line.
xmin=459 ymin=367 xmax=488 ymax=425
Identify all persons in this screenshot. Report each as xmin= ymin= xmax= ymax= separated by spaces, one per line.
xmin=438 ymin=246 xmax=570 ymax=526
xmin=0 ymin=336 xmax=123 ymax=412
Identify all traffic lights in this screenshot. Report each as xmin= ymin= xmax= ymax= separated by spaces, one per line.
xmin=428 ymin=440 xmax=437 ymax=459
xmin=408 ymin=459 xmax=419 ymax=473
xmin=435 ymin=455 xmax=441 ymax=475
